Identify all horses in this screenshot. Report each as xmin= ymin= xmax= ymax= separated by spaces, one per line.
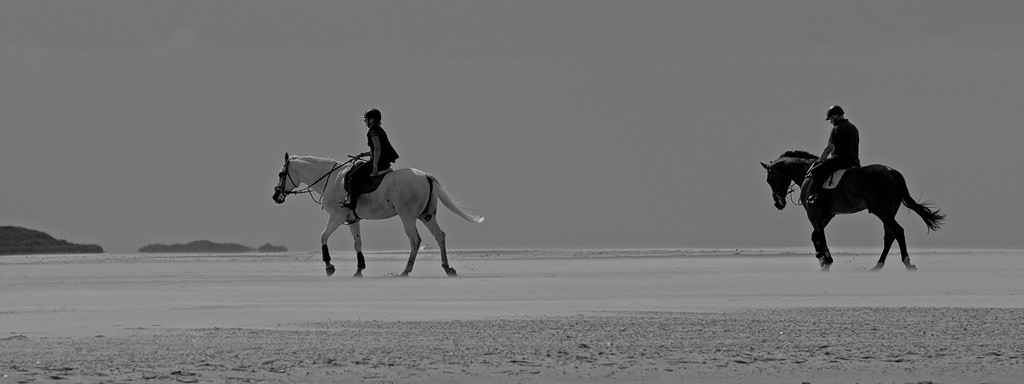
xmin=271 ymin=152 xmax=484 ymax=276
xmin=759 ymin=150 xmax=947 ymax=271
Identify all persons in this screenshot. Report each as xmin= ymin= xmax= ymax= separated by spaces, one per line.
xmin=803 ymin=103 xmax=860 ymax=206
xmin=339 ymin=108 xmax=400 ymax=211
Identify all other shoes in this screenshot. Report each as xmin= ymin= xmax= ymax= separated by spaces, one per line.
xmin=338 ymin=200 xmax=357 ymax=210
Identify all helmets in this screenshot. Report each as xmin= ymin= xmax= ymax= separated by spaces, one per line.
xmin=826 ymin=105 xmax=844 ymax=120
xmin=362 ymin=109 xmax=381 ymax=121
